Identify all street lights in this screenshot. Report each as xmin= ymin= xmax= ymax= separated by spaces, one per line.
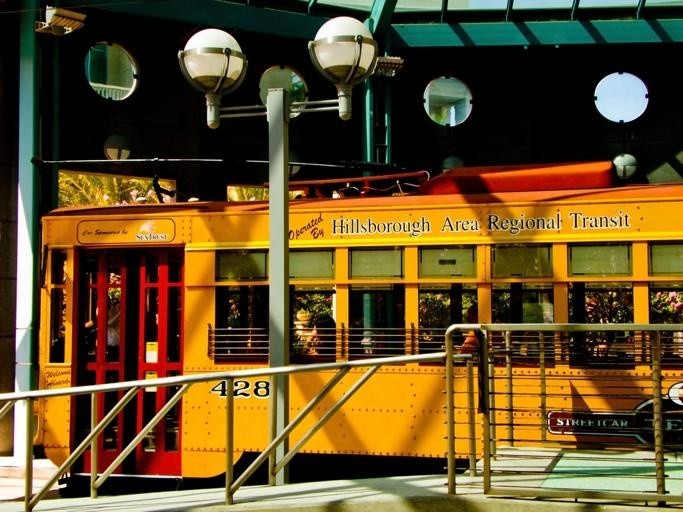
xmin=178 ymin=11 xmax=385 ymax=480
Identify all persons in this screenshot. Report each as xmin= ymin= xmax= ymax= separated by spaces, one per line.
xmin=302 ymin=313 xmax=336 ymax=364
xmin=52 ymin=299 xmax=120 ymax=362
xmin=457 ymin=304 xmax=478 ymax=353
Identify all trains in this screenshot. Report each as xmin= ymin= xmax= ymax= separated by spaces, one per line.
xmin=26 ymin=157 xmax=682 ymax=481
